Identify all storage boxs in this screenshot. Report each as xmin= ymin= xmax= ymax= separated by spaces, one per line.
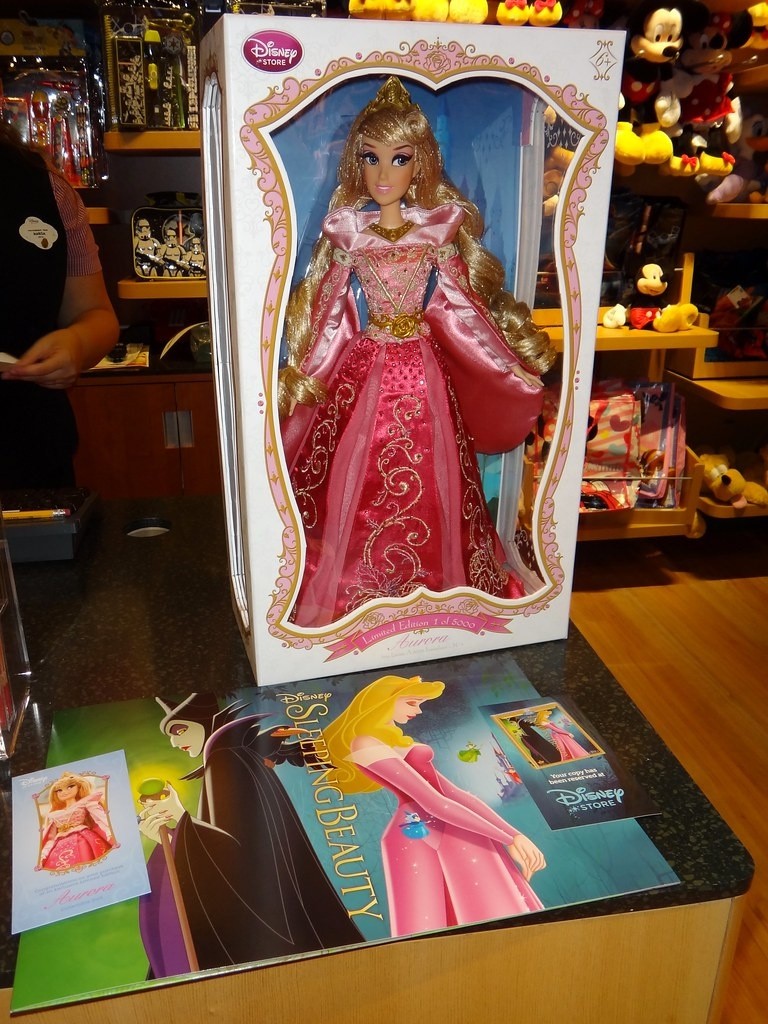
xmin=195 ymin=15 xmax=627 ymax=690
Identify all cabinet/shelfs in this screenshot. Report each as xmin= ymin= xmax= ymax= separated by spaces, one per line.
xmin=81 ymin=126 xmax=224 ymax=504
xmin=527 ymin=164 xmax=768 ymax=544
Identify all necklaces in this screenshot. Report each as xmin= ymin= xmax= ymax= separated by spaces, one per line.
xmin=370 ymin=221 xmax=414 ymax=242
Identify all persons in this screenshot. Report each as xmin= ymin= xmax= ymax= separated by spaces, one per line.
xmin=0 ymin=117 xmax=120 ymax=488
xmin=133 ymin=219 xmax=208 ymax=278
xmin=282 ymin=99 xmax=557 ymax=623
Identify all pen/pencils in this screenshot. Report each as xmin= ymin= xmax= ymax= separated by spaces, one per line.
xmin=2 ymin=508 xmax=73 ymax=520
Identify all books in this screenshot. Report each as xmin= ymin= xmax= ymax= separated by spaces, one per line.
xmin=2 ymin=487 xmax=90 ymax=521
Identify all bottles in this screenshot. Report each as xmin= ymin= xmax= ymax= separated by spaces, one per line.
xmin=144 ymin=29 xmax=164 ymax=126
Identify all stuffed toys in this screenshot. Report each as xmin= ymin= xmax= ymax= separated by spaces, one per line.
xmin=686 ymin=448 xmax=768 ymax=539
xmin=349 ymin=0 xmax=563 ymax=26
xmin=615 ymin=0 xmax=768 ymax=205
xmin=603 ymin=258 xmax=698 ymax=333
xmin=544 ymin=107 xmax=575 ymax=216
